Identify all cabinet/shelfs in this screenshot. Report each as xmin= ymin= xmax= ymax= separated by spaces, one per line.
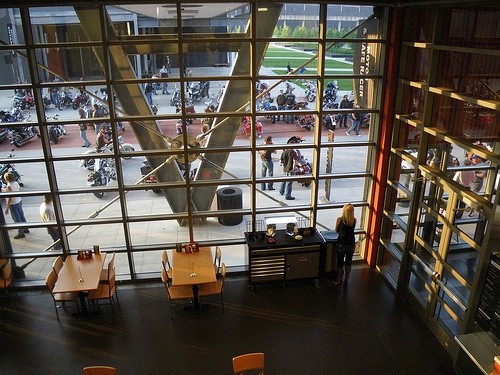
xmin=244 ymin=227 xmax=326 ymax=291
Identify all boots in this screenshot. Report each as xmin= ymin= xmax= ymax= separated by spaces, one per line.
xmin=343 ymin=271 xmax=350 ymax=288
xmin=334 ymin=273 xmax=343 ymax=286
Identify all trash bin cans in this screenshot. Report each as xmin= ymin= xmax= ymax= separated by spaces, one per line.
xmin=216 ymin=187 xmax=242 ymax=227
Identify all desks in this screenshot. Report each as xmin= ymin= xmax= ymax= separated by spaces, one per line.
xmin=172 ymin=246 xmax=217 ymax=312
xmin=52 ymin=252 xmax=105 ymax=315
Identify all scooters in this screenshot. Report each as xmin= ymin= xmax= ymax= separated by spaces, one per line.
xmin=241 ymin=78 xmax=372 ymax=140
xmin=0 ymin=148 xmax=24 ymax=191
xmin=294 ymin=139 xmax=313 ymax=187
xmin=0 ymin=76 xmax=226 ymax=198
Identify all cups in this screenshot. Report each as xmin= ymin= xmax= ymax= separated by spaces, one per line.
xmin=94 ymin=245 xmax=99 ymax=254
xmin=176 ymin=243 xmax=182 ymax=252
xmin=269 ymin=224 xmax=276 ymax=232
xmin=286 ymin=223 xmax=295 ymax=233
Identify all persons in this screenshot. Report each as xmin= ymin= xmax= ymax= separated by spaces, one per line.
xmin=3 ymin=172 xmax=29 ymax=239
xmin=255 ymin=80 xmax=295 ymax=124
xmin=201 ymin=81 xmax=210 ymax=98
xmin=331 ymin=204 xmax=356 ymax=289
xmin=143 ymin=55 xmax=172 ymax=105
xmin=279 ymin=141 xmax=296 ymax=200
xmin=183 ymin=65 xmax=193 ymax=92
xmin=92 ymin=90 xmax=114 ymax=153
xmin=40 ymin=194 xmax=63 ymax=250
xmin=339 ymin=95 xmax=351 ymax=128
xmin=78 ymin=109 xmax=92 ymax=147
xmin=16 ymin=77 xmax=62 ymax=111
xmin=78 ymin=77 xmax=86 ymax=95
xmin=285 ymin=61 xmax=306 ymax=85
xmin=430 ymin=143 xmax=489 ymax=220
xmin=345 ymin=104 xmax=363 ymax=136
xmin=258 ymin=135 xmax=277 ymax=191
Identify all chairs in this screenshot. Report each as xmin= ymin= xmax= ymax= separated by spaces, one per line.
xmin=0 ymin=259 xmax=18 ymax=303
xmin=46 ymin=252 xmax=120 ymax=323
xmin=233 ymin=353 xmax=264 ymax=375
xmin=161 ymin=248 xmax=228 ymax=318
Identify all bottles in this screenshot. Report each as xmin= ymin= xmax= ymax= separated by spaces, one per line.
xmin=185 ymin=242 xmax=199 ymax=253
xmin=78 ymin=250 xmax=92 ymax=259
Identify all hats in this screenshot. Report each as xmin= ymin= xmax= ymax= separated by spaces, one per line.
xmin=470 ymin=155 xmax=479 ymax=160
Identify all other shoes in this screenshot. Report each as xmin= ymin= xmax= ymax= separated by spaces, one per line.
xmin=24 ymin=230 xmax=29 ymax=233
xmin=83 ymin=143 xmax=91 ymax=148
xmin=14 ymin=234 xmax=25 ymax=239
xmin=286 ymin=196 xmax=295 ymax=200
xmin=356 ymin=134 xmax=361 ymax=136
xmin=346 ymin=131 xmax=350 ymax=136
